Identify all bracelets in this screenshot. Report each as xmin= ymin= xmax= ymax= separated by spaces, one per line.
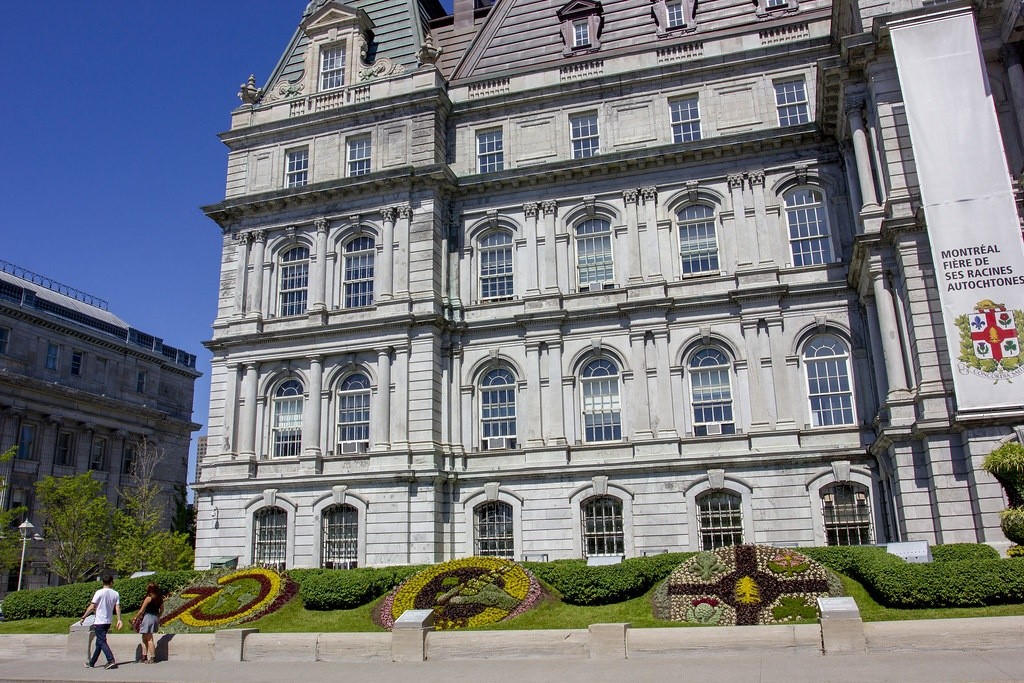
xmin=82 ymin=618 xmax=85 ymax=619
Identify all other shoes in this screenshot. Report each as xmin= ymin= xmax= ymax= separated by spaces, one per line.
xmin=105 ymin=657 xmax=115 ymax=669
xmin=136 ymin=655 xmax=148 ymax=663
xmin=144 ymin=657 xmax=156 ymax=664
xmin=83 ymin=662 xmax=94 ymax=668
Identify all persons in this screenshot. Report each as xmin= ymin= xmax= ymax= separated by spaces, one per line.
xmin=80 ymin=575 xmax=123 ymax=669
xmin=132 ymin=580 xmax=164 ymax=665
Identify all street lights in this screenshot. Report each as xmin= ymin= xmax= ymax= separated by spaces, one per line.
xmin=17 ymin=518 xmax=34 ymax=590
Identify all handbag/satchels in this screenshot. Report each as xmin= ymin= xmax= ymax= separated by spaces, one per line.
xmin=133 ymin=613 xmax=144 ymax=633
